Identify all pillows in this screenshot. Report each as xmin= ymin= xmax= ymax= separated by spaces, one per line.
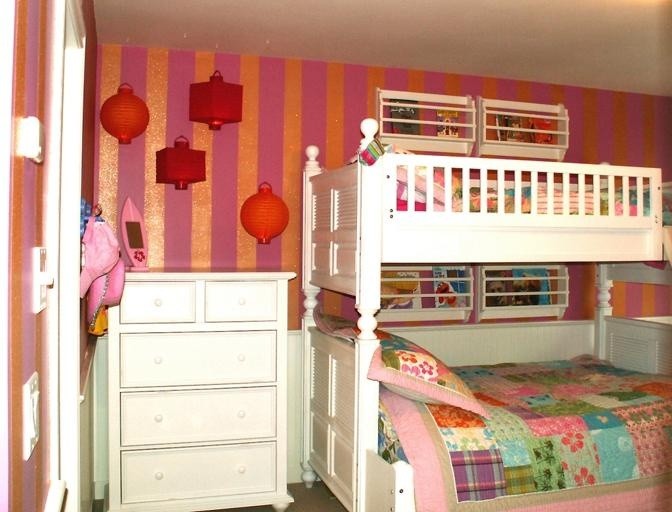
xmin=313 ymin=304 xmax=492 ymax=423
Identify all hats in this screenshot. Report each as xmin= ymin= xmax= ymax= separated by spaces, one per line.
xmin=87 ymin=256 xmax=126 ymax=328
xmin=80 ymin=220 xmax=124 ymax=296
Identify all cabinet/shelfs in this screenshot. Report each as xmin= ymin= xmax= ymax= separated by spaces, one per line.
xmin=93 ymin=271 xmax=297 ymax=511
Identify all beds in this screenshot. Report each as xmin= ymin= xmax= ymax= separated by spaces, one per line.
xmin=301 ymin=119 xmax=671 ymax=508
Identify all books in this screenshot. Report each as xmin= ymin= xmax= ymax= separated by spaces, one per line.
xmin=527 ymin=114 xmax=553 ymax=145
xmin=389 ymin=98 xmax=419 ymax=135
xmin=485 ymin=271 xmax=507 ymax=306
xmin=432 ymin=265 xmax=471 ymax=308
xmin=512 ymin=268 xmax=553 ymax=305
xmin=436 ymin=109 xmax=459 ymax=138
xmin=381 ymin=269 xmax=422 ymax=308
xmin=495 ymin=115 xmax=524 ymax=143
